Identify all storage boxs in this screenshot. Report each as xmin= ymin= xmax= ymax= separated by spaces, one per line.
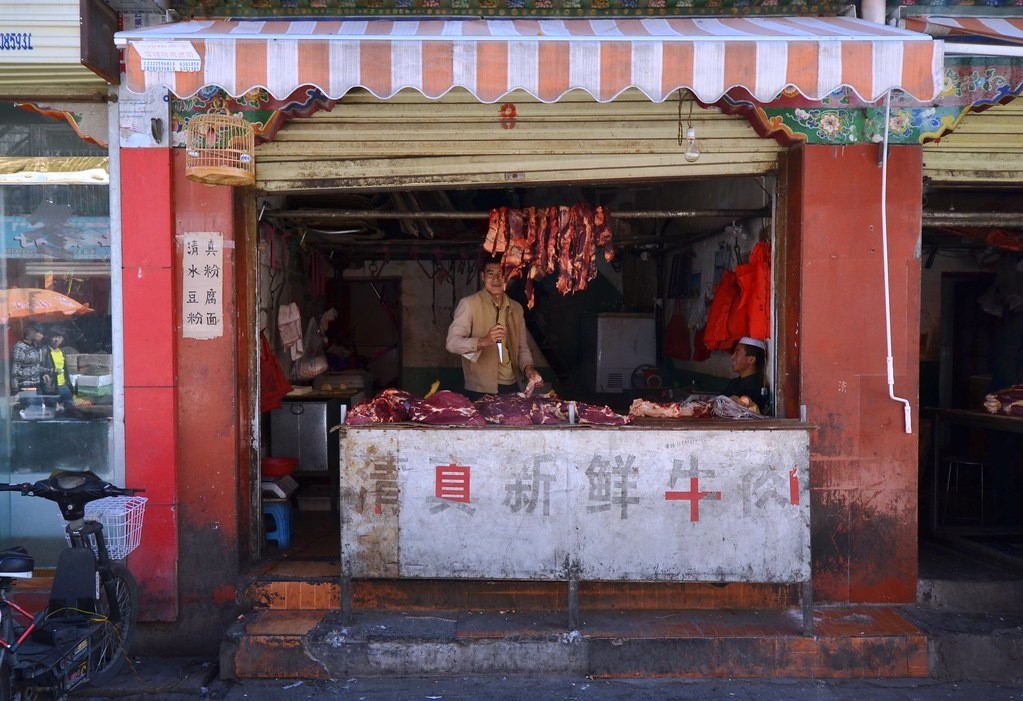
xmin=270 ymin=392 xmax=348 ymax=473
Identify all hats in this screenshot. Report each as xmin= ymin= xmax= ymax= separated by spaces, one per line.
xmin=738 ymin=337 xmax=764 ymax=350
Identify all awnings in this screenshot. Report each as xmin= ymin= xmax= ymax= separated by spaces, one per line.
xmin=903 ymin=14 xmax=1023 ymax=45
xmin=112 ymin=18 xmax=943 ymax=105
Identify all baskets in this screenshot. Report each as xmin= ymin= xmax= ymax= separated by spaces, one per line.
xmin=56 ymin=495 xmax=147 ymax=561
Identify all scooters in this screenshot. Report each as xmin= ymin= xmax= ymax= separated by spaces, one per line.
xmin=0 ymin=467 xmax=154 ymax=701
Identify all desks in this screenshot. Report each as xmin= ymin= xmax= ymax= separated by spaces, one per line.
xmin=339 ymin=422 xmax=814 ymax=638
xmin=923 ymin=405 xmax=1023 ymax=540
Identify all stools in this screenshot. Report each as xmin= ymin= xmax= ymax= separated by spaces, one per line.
xmin=944 ymin=454 xmax=999 ymax=529
xmin=263 ymin=498 xmax=293 ymax=549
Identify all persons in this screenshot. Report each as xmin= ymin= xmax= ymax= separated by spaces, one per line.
xmin=10 ymin=323 xmax=79 ymax=421
xmin=723 ymin=337 xmax=771 ymax=416
xmin=446 ymin=251 xmax=545 ymax=405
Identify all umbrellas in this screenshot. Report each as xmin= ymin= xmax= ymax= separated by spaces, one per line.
xmin=0 ymin=288 xmax=95 ymax=468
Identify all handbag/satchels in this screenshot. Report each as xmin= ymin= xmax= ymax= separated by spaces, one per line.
xmin=288 ymin=317 xmax=329 ymax=381
